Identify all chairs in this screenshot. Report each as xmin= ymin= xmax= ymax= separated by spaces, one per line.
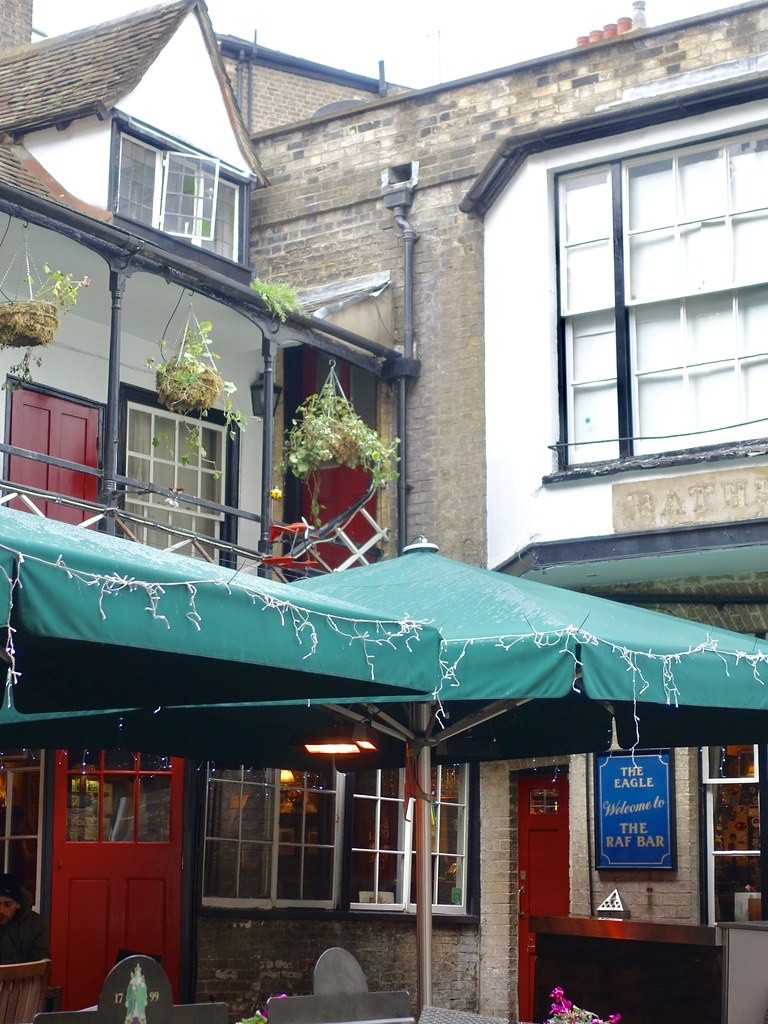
xmin=0 ymin=959 xmax=53 ymax=1024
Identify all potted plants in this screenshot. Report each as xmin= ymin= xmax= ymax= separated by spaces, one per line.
xmin=0 ymin=263 xmax=90 ymax=393
xmin=148 ymin=322 xmax=248 ymax=480
xmin=283 ymin=381 xmax=402 ymax=553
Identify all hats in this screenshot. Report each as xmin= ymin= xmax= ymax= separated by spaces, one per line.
xmin=0 ymin=874 xmax=24 ymax=904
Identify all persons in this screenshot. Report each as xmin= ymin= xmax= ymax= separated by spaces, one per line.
xmin=0 ymin=874 xmax=52 ymax=965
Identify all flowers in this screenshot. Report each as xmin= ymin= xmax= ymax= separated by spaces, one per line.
xmin=546 ymin=980 xmax=621 ymax=1024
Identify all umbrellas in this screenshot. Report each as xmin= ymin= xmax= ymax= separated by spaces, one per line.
xmin=0 ymin=505 xmax=442 ymax=736
xmin=105 ymin=535 xmax=768 ymax=1019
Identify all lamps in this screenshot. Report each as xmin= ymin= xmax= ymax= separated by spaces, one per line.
xmin=280 ymin=770 xmax=296 ymax=782
xmin=353 ymin=720 xmax=380 ymax=751
